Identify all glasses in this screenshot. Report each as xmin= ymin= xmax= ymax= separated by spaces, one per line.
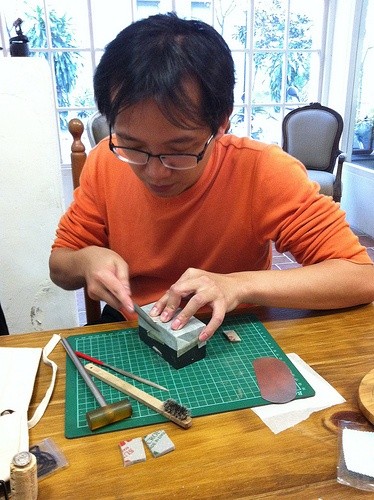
xmin=107 ymin=120 xmax=217 ymax=170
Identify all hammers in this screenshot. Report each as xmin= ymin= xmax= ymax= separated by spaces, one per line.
xmin=59 ymin=336 xmax=132 ymax=431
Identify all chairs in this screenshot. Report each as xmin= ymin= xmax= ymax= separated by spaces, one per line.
xmin=280 ymin=103 xmax=346 ymax=203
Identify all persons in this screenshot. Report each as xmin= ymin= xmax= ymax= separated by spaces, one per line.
xmin=48 ymin=10 xmax=374 ymax=342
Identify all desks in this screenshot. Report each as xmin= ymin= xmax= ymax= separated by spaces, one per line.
xmin=0 ymin=302 xmax=374 ymax=500
